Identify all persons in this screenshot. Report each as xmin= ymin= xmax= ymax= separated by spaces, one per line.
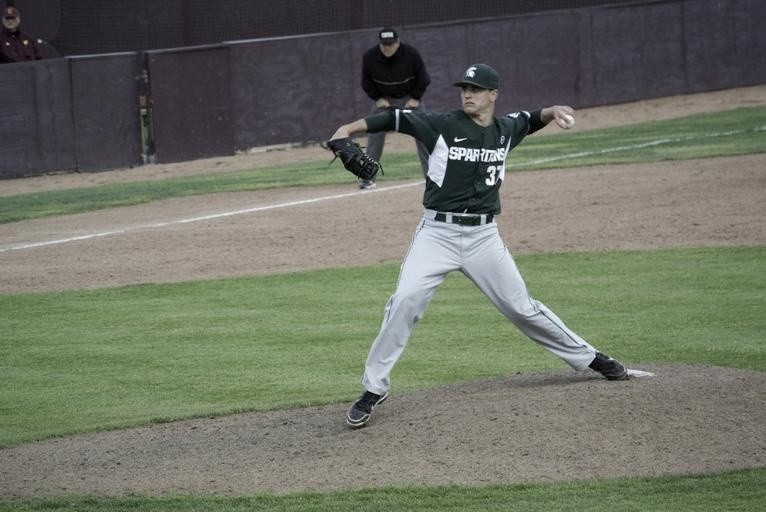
xmin=355 ymin=27 xmax=432 ymax=190
xmin=321 ymin=62 xmax=629 ymax=430
xmin=0 ymin=5 xmax=42 ymax=63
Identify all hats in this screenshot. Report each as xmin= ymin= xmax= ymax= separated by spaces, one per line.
xmin=379 ymin=28 xmax=398 ymax=44
xmin=453 ymin=64 xmax=500 ymax=90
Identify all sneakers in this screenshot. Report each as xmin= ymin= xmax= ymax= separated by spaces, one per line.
xmin=589 ymin=352 xmax=628 ymax=381
xmin=348 ymin=392 xmax=388 ymax=429
xmin=360 ymin=178 xmax=375 ymax=188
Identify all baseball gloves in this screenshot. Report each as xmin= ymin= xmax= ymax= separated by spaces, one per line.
xmin=326 ymin=138 xmax=383 ymax=181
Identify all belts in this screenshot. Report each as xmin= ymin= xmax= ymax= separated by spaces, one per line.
xmin=434 ymin=212 xmax=494 ymax=227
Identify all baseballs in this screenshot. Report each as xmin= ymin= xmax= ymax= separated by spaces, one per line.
xmin=559 ymin=113 xmax=573 ymax=128
